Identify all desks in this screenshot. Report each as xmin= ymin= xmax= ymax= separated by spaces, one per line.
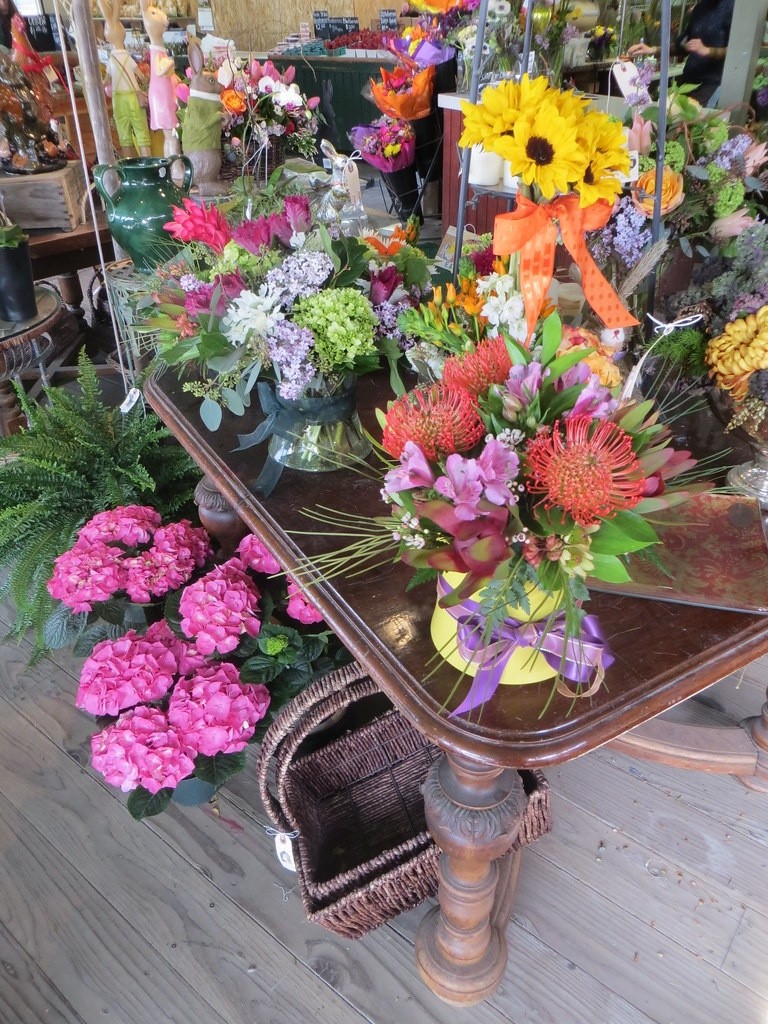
xmin=142 ymin=372 xmax=768 ymax=1003
xmin=0 ymin=286 xmax=64 ymax=417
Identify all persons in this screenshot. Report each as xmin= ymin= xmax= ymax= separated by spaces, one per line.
xmin=0 ymin=0 xmax=39 ymax=52
xmin=627 ymin=0 xmax=735 ymax=107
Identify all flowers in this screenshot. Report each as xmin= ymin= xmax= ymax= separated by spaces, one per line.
xmin=267 ymin=306 xmax=707 ymax=721
xmin=42 ymin=505 xmax=358 ymax=822
xmin=74 ymin=0 xmax=768 ymax=461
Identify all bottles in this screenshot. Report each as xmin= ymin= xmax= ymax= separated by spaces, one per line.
xmin=93 ymin=154 xmax=194 ymax=274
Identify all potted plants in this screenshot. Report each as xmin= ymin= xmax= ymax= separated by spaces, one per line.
xmin=0 ymin=345 xmax=204 ymax=624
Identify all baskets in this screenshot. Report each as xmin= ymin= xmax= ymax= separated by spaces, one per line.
xmin=219 ymin=134 xmax=286 ymax=182
xmin=256 ymin=657 xmax=551 ymax=939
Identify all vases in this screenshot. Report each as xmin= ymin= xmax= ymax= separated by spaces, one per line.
xmin=710 ymin=393 xmax=768 ymax=510
xmin=430 ymin=571 xmax=562 ymax=686
xmin=269 ymin=387 xmax=371 ymax=471
